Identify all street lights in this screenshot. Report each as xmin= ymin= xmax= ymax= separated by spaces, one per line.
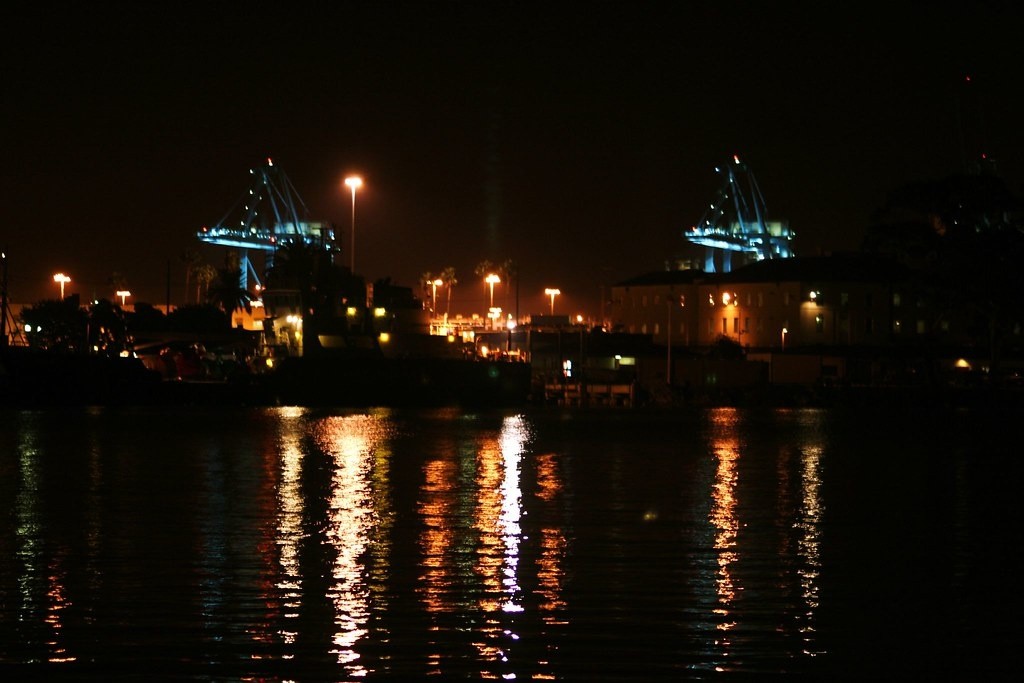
xmin=344 ymin=175 xmax=360 ymax=274
xmin=54 ymin=273 xmax=71 ymax=301
xmin=117 ymin=291 xmax=131 ymax=309
xmin=484 ymin=274 xmax=501 ymax=326
xmin=544 ymin=288 xmax=561 ymax=314
xmin=428 ymin=278 xmax=443 ymax=319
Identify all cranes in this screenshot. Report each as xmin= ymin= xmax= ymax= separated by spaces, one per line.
xmin=198 ymin=157 xmax=338 ymax=255
xmin=684 ymin=152 xmax=794 ymax=260
